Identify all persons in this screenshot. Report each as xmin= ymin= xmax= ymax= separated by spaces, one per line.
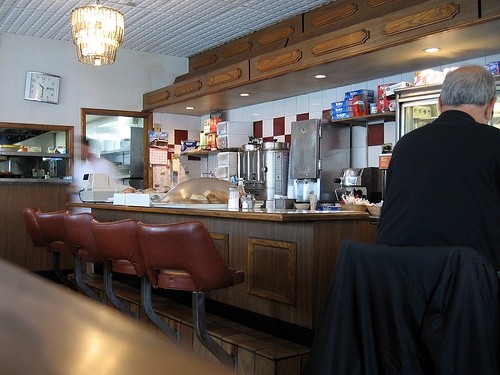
xmin=375 ymin=65 xmax=500 ymax=278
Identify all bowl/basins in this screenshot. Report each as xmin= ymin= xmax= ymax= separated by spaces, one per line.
xmin=275 ymin=199 xmax=296 ymax=209
xmin=341 ymin=204 xmax=367 ymax=212
xmin=295 ymin=204 xmax=311 ymax=209
xmin=366 ymin=205 xmax=381 ymax=218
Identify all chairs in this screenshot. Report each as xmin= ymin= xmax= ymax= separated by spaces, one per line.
xmin=338 ymin=239 xmax=493 ymax=374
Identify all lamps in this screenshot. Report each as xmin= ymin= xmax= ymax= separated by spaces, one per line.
xmin=71 ymin=0 xmax=125 ymax=65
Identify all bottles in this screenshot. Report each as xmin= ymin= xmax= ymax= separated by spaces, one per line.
xmin=370 ymin=103 xmax=378 ymax=114
xmin=241 ymin=194 xmax=254 ymax=209
xmin=228 ymin=187 xmax=240 ymax=212
xmin=266 ymin=200 xmax=275 ymax=213
xmin=353 ymin=94 xmax=368 ymax=116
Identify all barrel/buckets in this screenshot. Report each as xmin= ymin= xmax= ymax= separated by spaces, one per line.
xmin=239 ymin=139 xmax=289 ymax=204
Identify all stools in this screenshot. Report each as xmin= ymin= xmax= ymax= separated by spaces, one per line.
xmin=24 ymin=207 xmax=244 ymax=369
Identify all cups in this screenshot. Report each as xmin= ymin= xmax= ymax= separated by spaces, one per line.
xmin=309 ymin=194 xmax=318 ymax=210
xmin=48 ymin=147 xmax=53 ymax=153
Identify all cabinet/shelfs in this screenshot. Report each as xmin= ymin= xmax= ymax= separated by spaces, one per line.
xmin=0 ymin=153 xmax=70 ymax=182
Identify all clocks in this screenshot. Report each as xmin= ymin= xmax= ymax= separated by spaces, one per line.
xmin=25 ymin=71 xmax=60 ymax=104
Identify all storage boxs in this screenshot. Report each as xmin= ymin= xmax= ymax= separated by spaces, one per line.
xmin=484 ymin=60 xmax=500 ymax=76
xmin=330 ymin=81 xmax=413 ymax=119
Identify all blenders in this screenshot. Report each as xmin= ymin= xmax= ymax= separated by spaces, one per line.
xmin=334 ymin=167 xmax=381 ymax=203
xmin=286 ymin=118 xmax=351 ymax=204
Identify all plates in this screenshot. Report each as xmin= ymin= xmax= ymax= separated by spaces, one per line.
xmin=0 ymin=148 xmax=19 ymax=152
